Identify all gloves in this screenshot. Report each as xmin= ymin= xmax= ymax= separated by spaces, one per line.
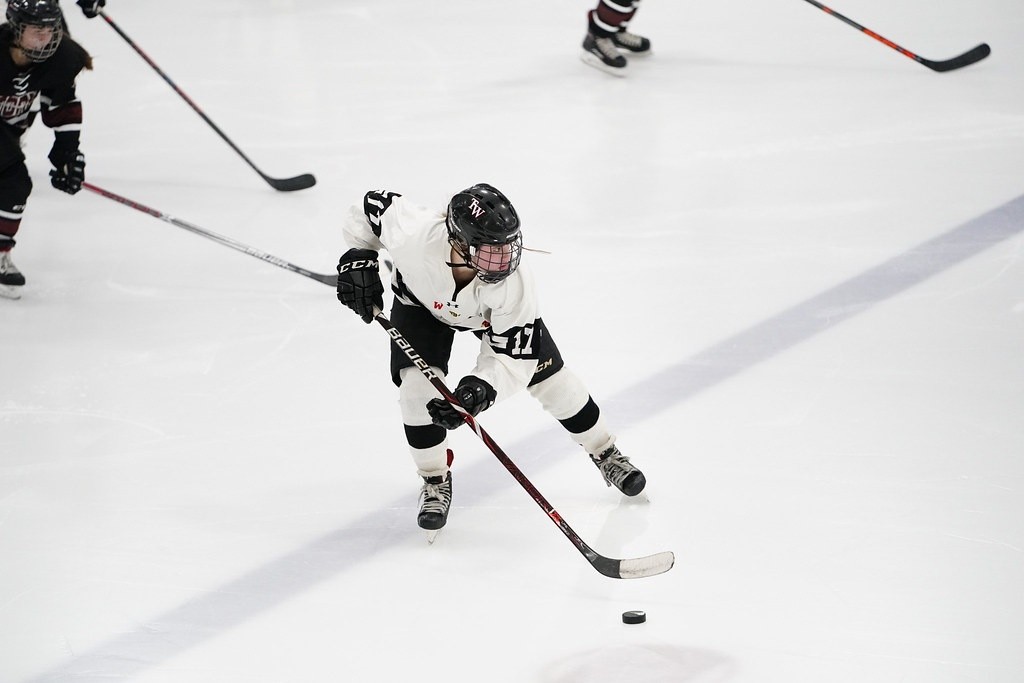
xmin=76 ymin=0 xmax=105 ymax=18
xmin=425 ymin=375 xmax=497 ymax=430
xmin=336 ymin=247 xmax=384 ymax=324
xmin=48 ymin=149 xmax=86 ymax=195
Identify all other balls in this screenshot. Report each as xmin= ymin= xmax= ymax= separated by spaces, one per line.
xmin=622 ymin=611 xmax=647 ymax=625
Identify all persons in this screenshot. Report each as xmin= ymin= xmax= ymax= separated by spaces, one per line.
xmin=576 ymin=0 xmax=651 ymax=77
xmin=337 ymin=184 xmax=646 ymax=544
xmin=0 ymin=0 xmax=106 ymax=301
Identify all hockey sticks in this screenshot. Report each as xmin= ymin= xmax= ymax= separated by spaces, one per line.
xmin=371 ymin=303 xmax=676 ymax=581
xmin=96 ymin=6 xmax=318 ymax=196
xmin=48 ymin=164 xmax=344 ymax=290
xmin=804 ymin=0 xmax=992 ymax=72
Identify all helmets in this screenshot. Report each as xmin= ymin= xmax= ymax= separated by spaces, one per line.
xmin=6 ymin=0 xmax=62 ymax=63
xmin=445 ymin=183 xmax=522 ymax=284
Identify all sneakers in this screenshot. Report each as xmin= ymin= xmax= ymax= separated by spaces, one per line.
xmin=611 ymin=25 xmax=651 ymax=56
xmin=416 ymin=449 xmax=454 ymax=543
xmin=0 ymin=240 xmax=26 ymax=300
xmin=579 ymin=434 xmax=651 ymax=504
xmin=580 ymin=10 xmax=628 ymax=77
